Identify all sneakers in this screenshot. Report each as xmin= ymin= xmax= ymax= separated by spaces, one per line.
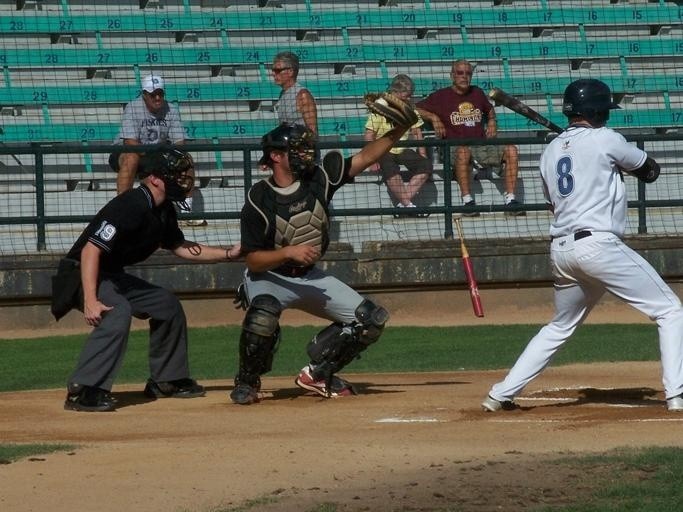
xmin=463 ymin=200 xmax=480 ymax=218
xmin=143 ymin=377 xmax=206 ymax=399
xmin=483 ymin=396 xmax=520 ymax=411
xmin=668 ymin=394 xmax=683 ymax=413
xmin=231 ymin=380 xmax=260 ymax=405
xmin=505 ymin=201 xmax=526 ymax=217
xmin=64 ymin=384 xmax=116 ymax=411
xmin=185 ymin=219 xmax=207 ymax=227
xmin=296 ymin=364 xmax=350 ymax=398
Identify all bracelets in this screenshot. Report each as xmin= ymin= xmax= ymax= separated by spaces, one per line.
xmin=419 ymin=152 xmax=426 ymax=156
xmin=225 ymin=249 xmax=232 ymax=261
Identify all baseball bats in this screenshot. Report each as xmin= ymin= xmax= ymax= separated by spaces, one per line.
xmin=454 ymin=215 xmax=484 ymax=318
xmin=489 ymin=88 xmax=565 ymax=133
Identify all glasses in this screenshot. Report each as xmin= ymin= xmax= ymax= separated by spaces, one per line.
xmin=271 ymin=66 xmax=290 ymax=73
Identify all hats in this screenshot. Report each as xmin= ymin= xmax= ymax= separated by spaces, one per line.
xmin=141 ymin=75 xmax=163 ymax=92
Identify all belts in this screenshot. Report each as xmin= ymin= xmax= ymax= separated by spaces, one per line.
xmin=277 ymin=268 xmax=309 ymax=277
xmin=574 ymin=231 xmax=591 ymax=239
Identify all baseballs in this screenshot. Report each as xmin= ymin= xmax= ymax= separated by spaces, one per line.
xmin=374 ymin=97 xmax=387 ymax=106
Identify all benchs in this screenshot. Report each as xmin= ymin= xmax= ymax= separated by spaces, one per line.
xmin=0 ymin=0 xmax=682 ymax=191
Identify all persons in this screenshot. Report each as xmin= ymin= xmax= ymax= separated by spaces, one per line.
xmin=257 ymin=51 xmax=319 ymax=172
xmin=109 ymin=75 xmax=207 ymax=227
xmin=364 ymin=75 xmax=433 ymax=219
xmin=50 ymin=144 xmax=241 ymax=411
xmin=415 ymin=58 xmax=526 ymax=218
xmin=229 ymin=93 xmax=421 ymax=403
xmin=480 ymin=78 xmax=681 ymax=412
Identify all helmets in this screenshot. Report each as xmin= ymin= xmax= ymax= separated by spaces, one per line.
xmin=257 ymin=123 xmax=317 ymax=175
xmin=563 ymin=79 xmax=611 ymax=123
xmin=135 ymin=144 xmax=194 ymax=202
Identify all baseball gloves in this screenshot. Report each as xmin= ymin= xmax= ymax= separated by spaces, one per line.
xmin=365 ymin=91 xmax=419 ymax=128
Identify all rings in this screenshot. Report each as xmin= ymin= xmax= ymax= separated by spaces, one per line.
xmin=313 ymin=253 xmax=317 ymax=258
